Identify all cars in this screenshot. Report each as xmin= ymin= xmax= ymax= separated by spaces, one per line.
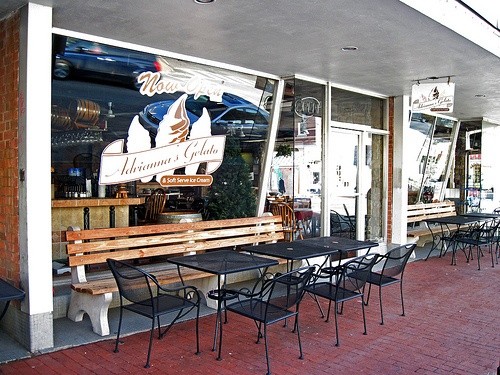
xmin=137 ymin=90 xmax=274 ymax=153
xmin=52 ymin=33 xmax=162 ymax=92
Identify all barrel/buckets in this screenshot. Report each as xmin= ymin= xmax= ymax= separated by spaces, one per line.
xmin=158 ymin=211 xmax=205 ymax=262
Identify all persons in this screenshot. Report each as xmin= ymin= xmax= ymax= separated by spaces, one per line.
xmin=279 ymin=177 xmax=286 ymax=195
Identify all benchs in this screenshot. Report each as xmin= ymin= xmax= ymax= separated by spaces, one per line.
xmin=68 ymin=214 xmax=285 ymax=336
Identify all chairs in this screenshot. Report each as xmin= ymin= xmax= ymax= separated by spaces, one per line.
xmin=427 ymin=222 xmax=500 ymax=270
xmin=273 ymin=202 xmax=303 ymax=242
xmin=291 ymin=253 xmax=380 ymax=347
xmin=340 ymin=243 xmax=417 ymax=325
xmin=143 ymin=188 xmax=166 ymax=224
xmin=106 ymin=259 xmax=200 ymax=368
xmin=208 ymin=265 xmax=316 ymax=375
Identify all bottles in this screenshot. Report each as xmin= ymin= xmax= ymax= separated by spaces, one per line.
xmin=64 ymin=185 xmax=91 ymax=198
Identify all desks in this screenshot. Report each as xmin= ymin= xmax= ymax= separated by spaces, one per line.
xmin=421 ymin=216 xmax=483 ymax=265
xmin=169 ymin=235 xmax=379 ymax=324
xmin=51 ymin=197 xmax=146 ymax=260
xmin=462 ymin=213 xmax=500 ymax=218
